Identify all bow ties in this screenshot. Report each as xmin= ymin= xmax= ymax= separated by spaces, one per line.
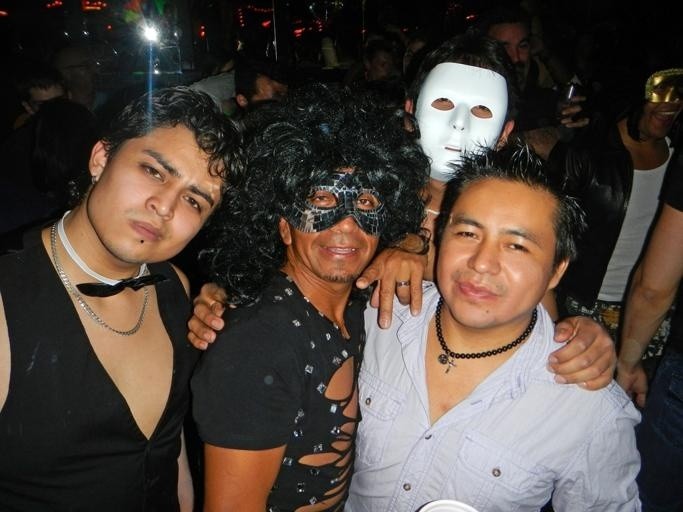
xmin=76 ymin=275 xmax=168 ymax=298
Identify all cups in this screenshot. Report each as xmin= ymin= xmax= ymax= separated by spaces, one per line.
xmin=554 ymin=81 xmax=585 ymax=121
